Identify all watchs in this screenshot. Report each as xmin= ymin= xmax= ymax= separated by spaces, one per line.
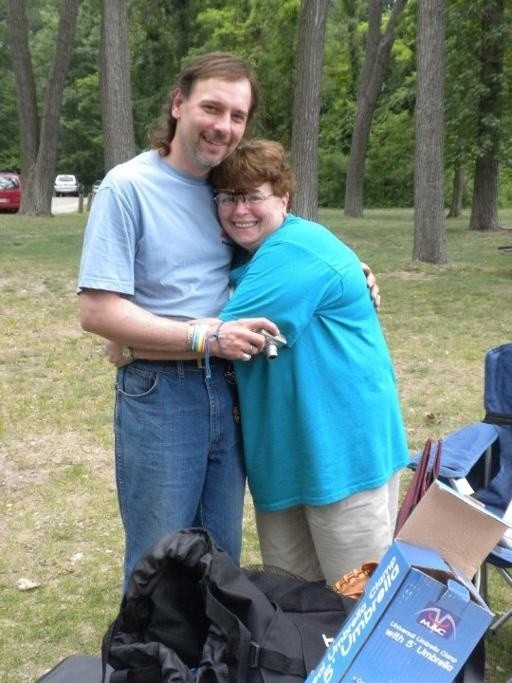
xmin=120 ymin=347 xmax=136 ymax=364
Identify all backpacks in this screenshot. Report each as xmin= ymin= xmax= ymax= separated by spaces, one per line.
xmin=101 ymin=525 xmax=358 ymax=683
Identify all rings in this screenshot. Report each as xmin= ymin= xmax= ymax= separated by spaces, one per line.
xmin=247 ymin=345 xmax=253 ymax=354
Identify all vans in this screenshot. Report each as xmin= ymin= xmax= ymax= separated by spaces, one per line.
xmin=0 ymin=173 xmax=24 ymax=212
xmin=53 ymin=174 xmax=79 ymax=197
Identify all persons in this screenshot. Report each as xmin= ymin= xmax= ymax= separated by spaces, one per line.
xmin=78 ymin=50 xmax=383 ymax=595
xmin=102 ymin=139 xmax=413 ymax=593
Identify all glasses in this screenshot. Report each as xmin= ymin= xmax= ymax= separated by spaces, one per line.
xmin=214 ymin=192 xmax=275 ymax=207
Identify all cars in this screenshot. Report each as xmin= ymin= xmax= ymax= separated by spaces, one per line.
xmin=91 ymin=179 xmax=102 ymax=193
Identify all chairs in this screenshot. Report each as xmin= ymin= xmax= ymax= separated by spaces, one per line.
xmin=411 ymin=341 xmax=512 ymax=633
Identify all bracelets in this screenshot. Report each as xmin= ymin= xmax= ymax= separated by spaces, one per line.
xmin=185 ymin=320 xmax=209 ymax=353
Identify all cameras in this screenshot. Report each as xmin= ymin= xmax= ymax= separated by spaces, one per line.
xmin=256 ymin=328 xmax=287 ymax=360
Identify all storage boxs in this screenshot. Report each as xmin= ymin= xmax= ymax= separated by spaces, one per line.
xmin=302 ymin=479 xmax=512 ymax=683
